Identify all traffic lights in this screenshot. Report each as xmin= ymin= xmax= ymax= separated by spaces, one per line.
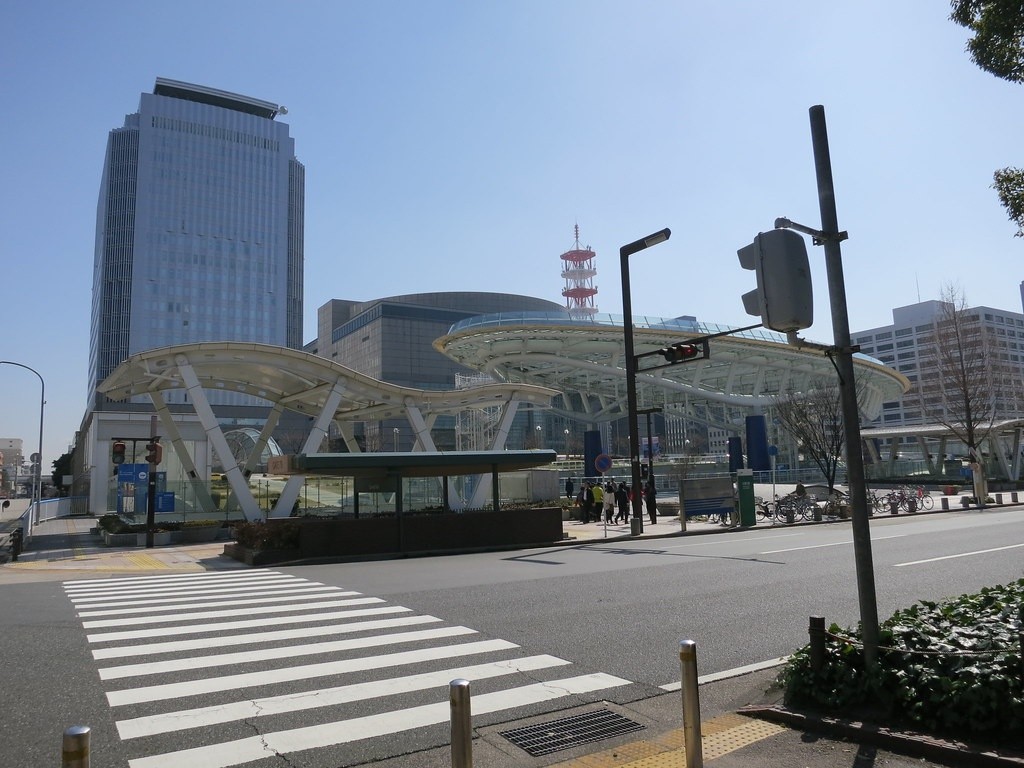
xmin=657 ymin=344 xmax=697 ymax=362
xmin=737 ymin=231 xmax=813 ymax=332
xmin=145 ymin=443 xmax=163 ymax=465
xmin=112 ymin=442 xmax=125 ymax=463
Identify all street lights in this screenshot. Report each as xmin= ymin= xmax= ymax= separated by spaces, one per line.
xmin=617 ymin=227 xmax=673 ymax=532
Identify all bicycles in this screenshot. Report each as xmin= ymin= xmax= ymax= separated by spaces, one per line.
xmin=878 ymin=492 xmax=916 ymax=512
xmin=753 ymin=502 xmax=792 ymax=522
xmin=905 ymin=485 xmax=934 ymax=510
xmin=890 ymin=492 xmax=924 ymax=510
xmin=829 ymin=488 xmax=884 ymax=517
xmin=763 ymin=494 xmax=823 ymax=522
xmin=712 ymin=511 xmax=734 ymax=525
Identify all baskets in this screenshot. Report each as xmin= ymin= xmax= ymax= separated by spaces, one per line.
xmin=922 ymin=489 xmax=930 ymax=495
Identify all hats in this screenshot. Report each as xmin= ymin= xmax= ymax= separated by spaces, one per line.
xmin=597 ymin=482 xmax=603 ymax=486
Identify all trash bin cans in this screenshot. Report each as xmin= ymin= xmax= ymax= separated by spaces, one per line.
xmin=960 ymin=468 xmax=967 ymax=476
xmin=965 ymin=469 xmax=972 ymax=481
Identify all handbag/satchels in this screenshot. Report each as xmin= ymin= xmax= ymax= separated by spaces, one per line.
xmin=609 ymin=504 xmax=614 ymax=512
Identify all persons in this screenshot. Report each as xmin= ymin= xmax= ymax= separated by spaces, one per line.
xmin=629 ymin=480 xmax=656 ymax=521
xmin=565 ymin=477 xmax=574 ymax=498
xmin=576 ymin=478 xmax=617 ymax=524
xmin=791 ymin=479 xmax=806 ymax=514
xmin=614 ymin=480 xmax=631 ymax=524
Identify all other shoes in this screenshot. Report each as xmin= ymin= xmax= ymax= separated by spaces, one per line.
xmin=605 ymin=519 xmax=629 ymax=525
